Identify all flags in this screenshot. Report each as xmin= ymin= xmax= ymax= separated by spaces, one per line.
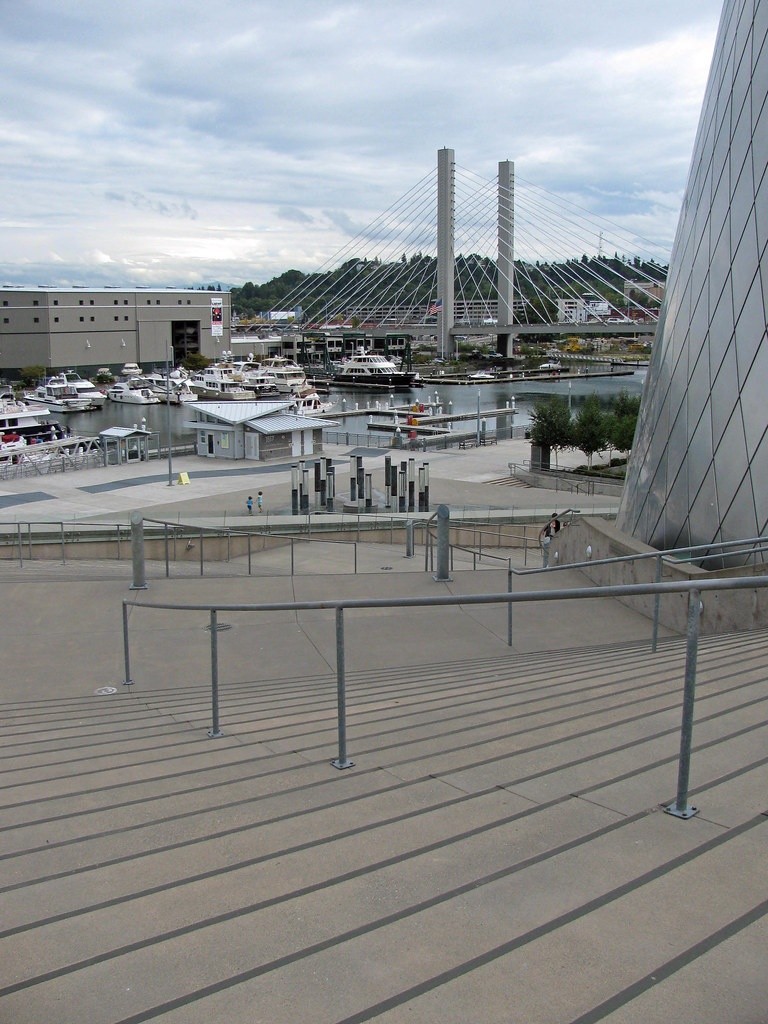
xmin=430 ymin=298 xmax=442 ymax=315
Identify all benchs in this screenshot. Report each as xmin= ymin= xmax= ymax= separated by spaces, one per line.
xmin=481 ymin=437 xmax=498 ymax=446
xmin=459 ymin=440 xmax=478 ymax=449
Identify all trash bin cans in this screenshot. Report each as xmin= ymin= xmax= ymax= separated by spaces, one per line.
xmin=410 ymin=431 xmax=416 ymax=440
xmin=130 ymin=450 xmax=144 ymax=461
xmin=420 ymin=404 xmax=424 ymax=413
xmin=406 ymin=415 xmax=413 ymax=425
xmin=525 ymin=432 xmax=531 ymax=439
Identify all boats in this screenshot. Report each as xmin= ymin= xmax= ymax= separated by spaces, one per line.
xmin=0 ymin=351 xmax=337 ymax=464
xmin=333 ymin=354 xmax=418 ymax=389
xmin=540 ymin=359 xmax=568 ymax=371
xmin=467 ymin=370 xmax=495 ymax=381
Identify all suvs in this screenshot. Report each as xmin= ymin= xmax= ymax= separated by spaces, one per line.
xmin=607 ymin=318 xmax=624 ymax=324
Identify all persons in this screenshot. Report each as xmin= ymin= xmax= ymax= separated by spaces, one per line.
xmin=543 ymin=513 xmax=560 ymax=537
xmin=254 ymin=491 xmax=263 ymax=513
xmin=246 ymin=496 xmax=253 ymax=514
